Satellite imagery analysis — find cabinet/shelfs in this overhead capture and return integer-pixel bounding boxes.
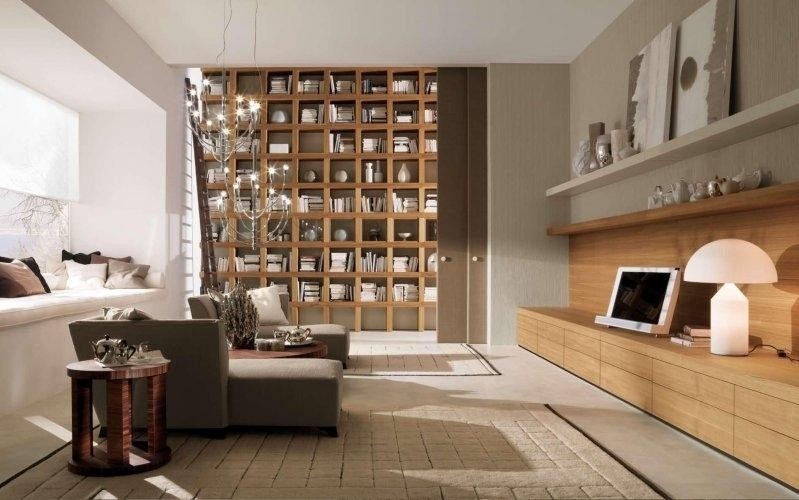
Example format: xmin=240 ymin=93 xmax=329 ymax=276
xmin=197 ymin=68 xmax=436 ymax=335
xmin=515 ymin=184 xmax=799 ymax=495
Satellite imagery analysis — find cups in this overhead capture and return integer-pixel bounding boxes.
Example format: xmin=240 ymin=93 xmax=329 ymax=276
xmin=137 ymin=342 xmax=152 ymax=361
xmin=257 ymin=339 xmax=284 ymax=352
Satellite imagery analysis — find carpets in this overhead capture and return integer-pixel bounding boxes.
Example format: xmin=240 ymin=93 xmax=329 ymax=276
xmin=336 ymin=338 xmax=502 ymax=377
xmin=0 ymin=402 xmax=663 ymax=499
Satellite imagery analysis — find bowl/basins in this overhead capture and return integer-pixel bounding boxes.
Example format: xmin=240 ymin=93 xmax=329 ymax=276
xmin=398 ymin=233 xmax=412 ymax=238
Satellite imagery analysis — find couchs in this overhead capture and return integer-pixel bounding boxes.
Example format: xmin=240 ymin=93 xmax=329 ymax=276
xmin=188 ymin=294 xmax=350 ymax=369
xmin=70 ymin=308 xmax=345 ymax=436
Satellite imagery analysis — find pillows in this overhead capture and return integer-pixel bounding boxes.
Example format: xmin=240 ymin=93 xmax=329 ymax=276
xmin=0 ymin=250 xmax=163 ymax=299
xmin=246 ymin=285 xmax=289 ymax=325
xmin=101 ymin=308 xmax=150 ymax=320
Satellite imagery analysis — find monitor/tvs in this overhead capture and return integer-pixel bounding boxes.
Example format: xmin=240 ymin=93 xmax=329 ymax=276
xmin=594 ymin=267 xmax=682 ymax=338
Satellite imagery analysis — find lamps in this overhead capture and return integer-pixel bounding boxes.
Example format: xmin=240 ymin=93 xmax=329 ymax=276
xmin=683 ymin=237 xmax=778 ymax=355
xmin=182 ymin=1 xmax=293 ymax=250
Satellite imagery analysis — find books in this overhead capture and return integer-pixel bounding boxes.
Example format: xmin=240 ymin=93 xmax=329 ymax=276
xmin=201 ymin=134 xmax=260 ymax=153
xmin=240 ymin=103 xmax=436 ymax=123
xmin=209 ymin=76 xmax=436 ymax=93
xmin=207 ymin=168 xmax=260 ymax=181
xmin=217 ymin=252 xmax=417 ymax=272
xmin=209 ymin=192 xmax=436 ymax=213
xmin=682 ymin=324 xmax=711 ymax=338
xmin=211 ymin=218 xmax=229 ymax=242
xmin=225 ymin=281 xmax=436 ymax=301
xmin=329 ymin=133 xmax=436 ymax=152
xmin=676 ymin=333 xmax=711 ymax=342
xmin=670 ymin=336 xmax=711 ymax=348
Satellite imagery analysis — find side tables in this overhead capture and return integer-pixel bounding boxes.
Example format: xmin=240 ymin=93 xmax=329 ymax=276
xmin=65 ymin=349 xmax=172 ymax=476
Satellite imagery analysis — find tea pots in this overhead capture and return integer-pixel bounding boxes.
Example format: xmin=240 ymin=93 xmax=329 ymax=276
xmin=89 ymin=336 xmax=133 ymax=365
xmin=647 ymin=168 xmax=761 ymax=211
xmin=272 ymin=326 xmax=311 ymax=344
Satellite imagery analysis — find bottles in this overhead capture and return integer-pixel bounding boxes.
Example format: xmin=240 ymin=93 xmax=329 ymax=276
xmin=571 ymin=127 xmax=632 ymax=178
xmin=365 ymin=160 xmax=384 ymax=182
xmin=268 ymin=230 xmax=291 ymax=242
xmin=397 ymin=163 xmax=412 ymax=182
xmin=328 ymin=104 xmax=336 ymax=123
